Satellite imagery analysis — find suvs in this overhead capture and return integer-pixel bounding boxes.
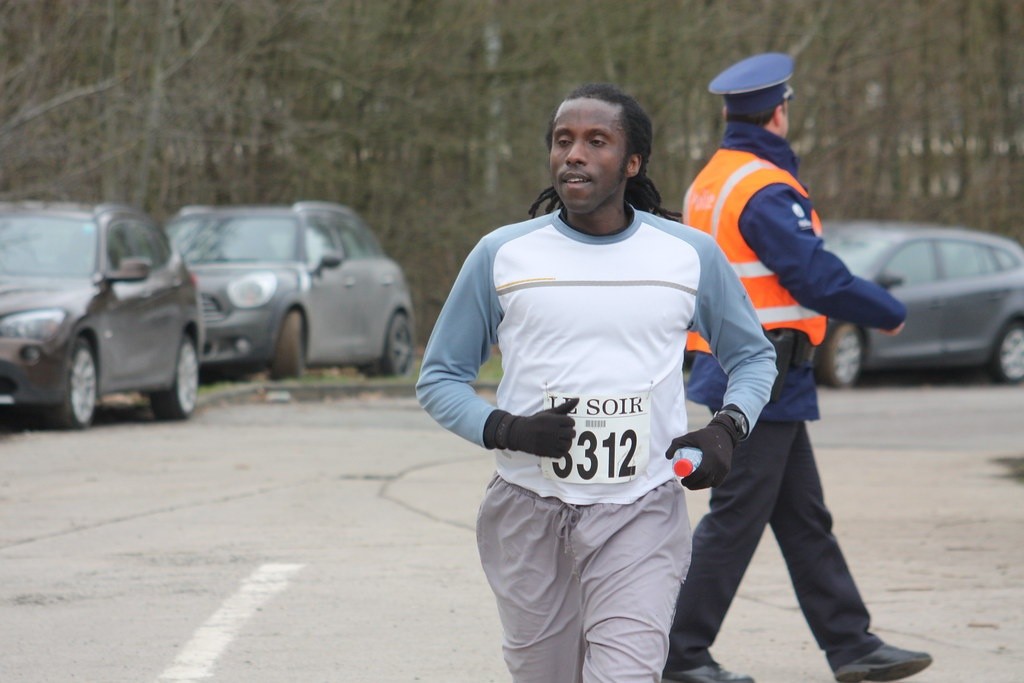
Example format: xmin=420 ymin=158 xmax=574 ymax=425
xmin=155 ymin=200 xmax=420 ymax=385
xmin=0 ymin=201 xmax=209 ymax=430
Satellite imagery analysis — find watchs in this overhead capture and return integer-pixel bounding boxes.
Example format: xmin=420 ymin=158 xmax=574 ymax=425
xmin=713 ymin=410 xmax=748 ymax=439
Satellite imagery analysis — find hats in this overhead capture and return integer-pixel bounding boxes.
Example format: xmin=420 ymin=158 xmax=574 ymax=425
xmin=708 ymin=53 xmax=795 ymax=113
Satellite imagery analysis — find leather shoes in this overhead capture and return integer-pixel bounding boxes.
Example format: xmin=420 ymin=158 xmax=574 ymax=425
xmin=834 ymin=643 xmax=932 ymax=683
xmin=662 ymin=663 xmax=754 ymax=683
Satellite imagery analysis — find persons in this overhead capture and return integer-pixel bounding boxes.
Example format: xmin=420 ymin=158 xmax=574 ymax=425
xmin=415 ymin=78 xmax=780 ymax=683
xmin=663 ymin=53 xmax=934 ymax=683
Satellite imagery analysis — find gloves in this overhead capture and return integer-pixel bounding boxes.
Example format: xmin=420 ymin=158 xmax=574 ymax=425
xmin=665 ymin=414 xmax=739 ymax=491
xmin=485 ymin=398 xmax=579 ymax=459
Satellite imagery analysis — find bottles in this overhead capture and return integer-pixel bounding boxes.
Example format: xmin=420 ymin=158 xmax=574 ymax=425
xmin=671 ymin=447 xmax=704 ymax=479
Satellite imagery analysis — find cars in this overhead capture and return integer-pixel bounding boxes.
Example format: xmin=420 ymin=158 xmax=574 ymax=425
xmin=808 ymin=223 xmax=1024 ymax=386
